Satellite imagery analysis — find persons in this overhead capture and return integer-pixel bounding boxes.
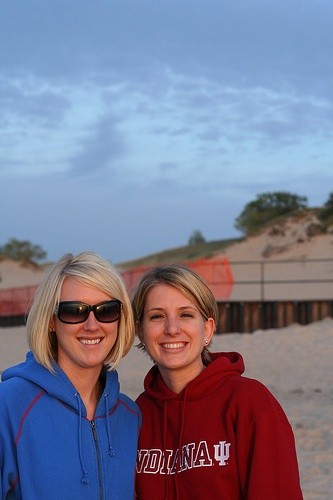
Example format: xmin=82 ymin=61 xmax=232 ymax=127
xmin=0 ymin=251 xmax=143 ymax=500
xmin=130 ymin=265 xmax=303 ymax=500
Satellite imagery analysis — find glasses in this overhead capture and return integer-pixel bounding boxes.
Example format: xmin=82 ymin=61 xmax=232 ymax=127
xmin=54 ymin=299 xmax=120 ymax=324
